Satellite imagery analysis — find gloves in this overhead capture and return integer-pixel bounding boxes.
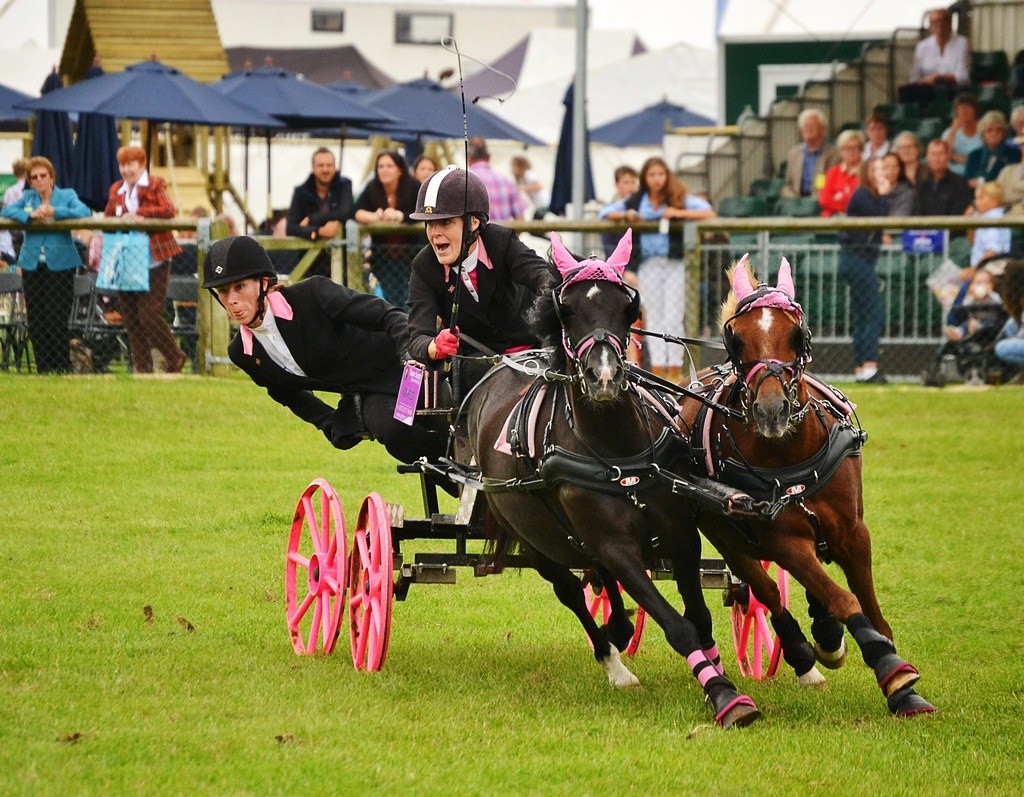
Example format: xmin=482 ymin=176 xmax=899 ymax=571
xmin=434 ymin=326 xmax=460 ymax=359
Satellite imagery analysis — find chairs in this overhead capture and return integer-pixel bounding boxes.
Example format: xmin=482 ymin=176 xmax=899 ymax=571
xmin=0 ymin=233 xmax=202 ymax=373
xmin=719 ymin=50 xmax=1024 ymax=327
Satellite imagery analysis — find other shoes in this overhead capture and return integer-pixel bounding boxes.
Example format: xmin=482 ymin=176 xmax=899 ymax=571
xmin=994 ymin=364 xmax=1023 ymax=387
xmin=854 ymin=365 xmax=878 ymax=382
xmin=168 ymin=351 xmax=188 ymax=372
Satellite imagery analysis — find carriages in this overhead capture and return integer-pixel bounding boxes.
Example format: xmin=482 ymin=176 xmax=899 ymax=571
xmin=283 ymin=228 xmax=941 ymax=733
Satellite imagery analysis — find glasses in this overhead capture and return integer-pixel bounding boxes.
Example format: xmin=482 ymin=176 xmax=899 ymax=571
xmin=30 ymin=173 xmax=49 ymax=180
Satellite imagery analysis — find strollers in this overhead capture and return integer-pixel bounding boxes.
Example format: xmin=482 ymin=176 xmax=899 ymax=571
xmin=920 ymin=251 xmax=1024 ymax=388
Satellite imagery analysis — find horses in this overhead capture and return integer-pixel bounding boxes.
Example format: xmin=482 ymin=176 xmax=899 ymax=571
xmin=465 ymin=227 xmax=814 ymax=732
xmin=673 ymin=251 xmax=937 ymax=716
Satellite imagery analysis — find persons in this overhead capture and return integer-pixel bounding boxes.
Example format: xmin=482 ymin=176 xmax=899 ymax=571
xmin=782 ymin=9 xmax=1024 ymax=388
xmin=406 ymin=165 xmax=558 ymax=397
xmin=601 ymin=156 xmax=715 ymax=380
xmin=257 ymin=137 xmax=546 ymax=307
xmin=0 ymin=155 xmax=93 ymax=374
xmin=100 ymin=145 xmax=187 ymax=372
xmin=201 ymin=235 xmax=463 ymax=497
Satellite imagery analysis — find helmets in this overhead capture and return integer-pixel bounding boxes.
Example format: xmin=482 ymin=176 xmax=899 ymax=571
xmin=200 ymin=235 xmax=277 ymax=289
xmin=410 ymin=163 xmax=490 ymax=222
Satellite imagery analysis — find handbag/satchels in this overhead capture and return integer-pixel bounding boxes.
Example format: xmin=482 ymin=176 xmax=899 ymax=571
xmin=95 ymin=229 xmax=153 ymax=294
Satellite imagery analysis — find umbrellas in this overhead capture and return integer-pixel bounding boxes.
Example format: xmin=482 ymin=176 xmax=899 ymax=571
xmin=1 ymin=54 xmax=720 ymax=216
